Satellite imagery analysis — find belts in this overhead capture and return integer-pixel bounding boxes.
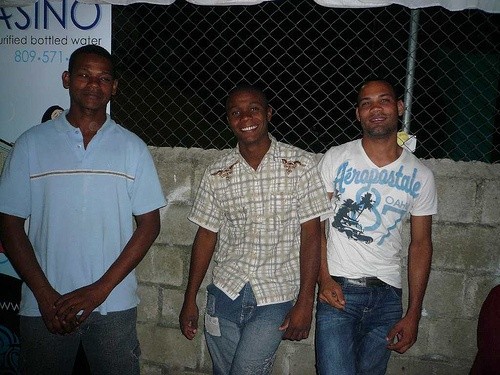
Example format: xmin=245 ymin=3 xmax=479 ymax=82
xmin=331 ymin=275 xmax=387 ymax=287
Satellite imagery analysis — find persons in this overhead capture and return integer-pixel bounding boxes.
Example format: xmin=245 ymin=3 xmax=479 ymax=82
xmin=0 ymin=44 xmax=167 ymax=375
xmin=179 ymin=84 xmax=334 ymax=375
xmin=316 ymin=81 xmax=438 ymax=375
